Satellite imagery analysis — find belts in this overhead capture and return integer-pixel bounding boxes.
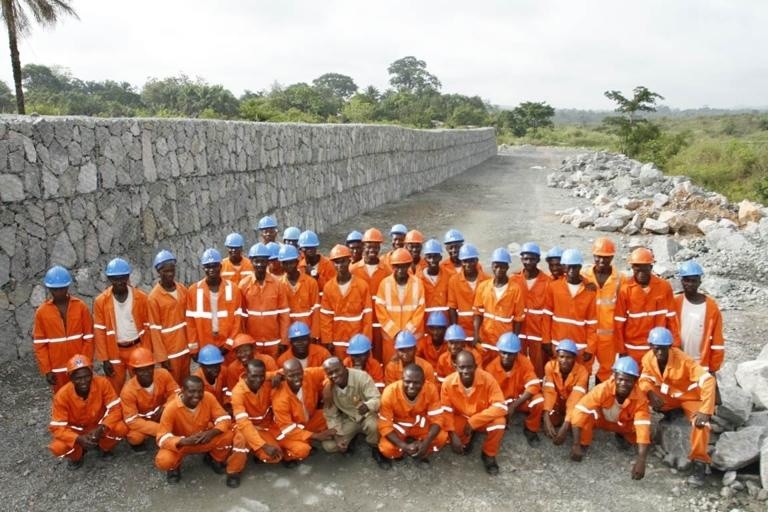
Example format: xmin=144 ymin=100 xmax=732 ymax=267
xmin=118 ymin=337 xmax=140 ymax=348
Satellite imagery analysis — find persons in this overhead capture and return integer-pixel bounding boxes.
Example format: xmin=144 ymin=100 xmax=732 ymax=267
xmin=32 ymin=215 xmax=727 ymax=492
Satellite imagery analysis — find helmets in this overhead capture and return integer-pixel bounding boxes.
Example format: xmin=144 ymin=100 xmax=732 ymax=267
xmin=66 ymin=354 xmax=94 ymax=377
xmin=329 ymin=224 xmax=480 ymax=265
xmin=496 ymin=331 xmax=521 ymax=354
xmin=628 ymin=247 xmax=655 ymax=265
xmin=224 ymin=233 xmax=244 ymax=248
xmin=43 ymin=265 xmax=73 ymax=289
xmin=346 ymin=334 xmax=371 ymax=355
xmin=647 ymin=326 xmax=674 ymax=346
xmin=491 ymin=248 xmax=512 ymax=263
xmin=105 ymin=258 xmax=131 ymax=276
xmin=592 ymin=237 xmax=615 ymax=257
xmin=198 ymin=344 xmax=225 ymax=366
xmin=611 ymin=356 xmax=640 ymax=378
xmin=231 ymin=332 xmax=256 ymax=352
xmin=679 ymin=262 xmax=703 ymax=277
xmin=200 ymin=248 xmax=222 ymax=265
xmin=129 ymin=347 xmax=155 ymax=368
xmin=153 ymin=249 xmax=176 ymax=270
xmin=520 ymin=242 xmax=584 ymax=265
xmin=394 ymin=311 xmax=466 ymax=349
xmin=555 ymin=338 xmax=579 ymax=357
xmin=248 ymin=216 xmax=320 ymax=261
xmin=287 ymin=320 xmax=310 ymax=339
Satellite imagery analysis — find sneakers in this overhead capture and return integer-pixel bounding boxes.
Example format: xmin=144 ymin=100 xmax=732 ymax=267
xmin=166 ymin=467 xmax=180 ymax=483
xmin=67 ymin=455 xmax=83 ymax=470
xmin=615 ymin=432 xmax=638 ymax=455
xmin=463 ymin=430 xmax=475 ymax=456
xmin=95 ymin=444 xmax=114 ymax=460
xmin=523 ymin=427 xmax=540 ymax=448
xmin=342 ymin=438 xmax=355 ymax=459
xmin=227 ymin=472 xmax=241 ymax=488
xmin=481 ymin=451 xmax=499 ymax=475
xmin=687 ymin=460 xmax=713 ymax=487
xmin=130 ymin=443 xmax=148 ymax=455
xmin=371 ymin=446 xmax=392 ymax=470
xmin=215 ymin=462 xmax=226 ymax=474
xmin=280 ymin=459 xmax=297 ymax=468
